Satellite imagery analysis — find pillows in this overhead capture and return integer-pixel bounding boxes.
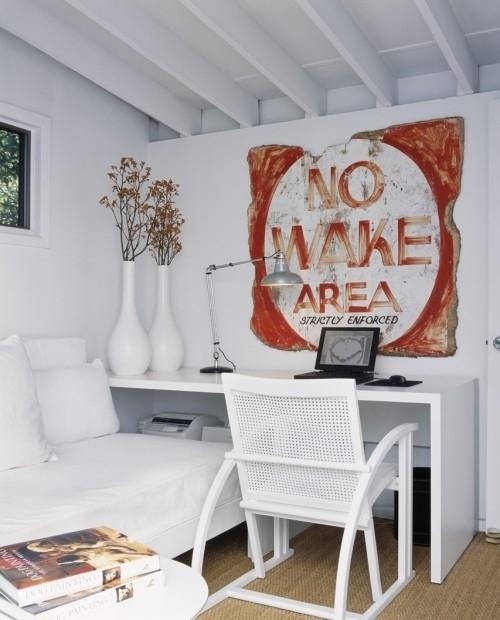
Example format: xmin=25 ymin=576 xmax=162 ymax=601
xmin=34 ymin=359 xmax=119 ymax=445
xmin=23 ymin=338 xmax=86 ymax=369
xmin=0 ymin=334 xmax=57 ymax=471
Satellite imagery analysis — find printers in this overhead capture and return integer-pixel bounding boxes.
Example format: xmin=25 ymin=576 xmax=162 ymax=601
xmin=137 ymin=411 xmax=225 ymax=441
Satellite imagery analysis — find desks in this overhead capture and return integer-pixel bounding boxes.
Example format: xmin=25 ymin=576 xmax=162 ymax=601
xmin=108 ymin=369 xmax=478 ymax=584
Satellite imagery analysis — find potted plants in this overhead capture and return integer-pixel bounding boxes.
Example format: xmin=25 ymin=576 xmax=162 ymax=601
xmin=142 ymin=177 xmax=183 ymax=371
xmin=100 ymin=157 xmax=151 ymax=377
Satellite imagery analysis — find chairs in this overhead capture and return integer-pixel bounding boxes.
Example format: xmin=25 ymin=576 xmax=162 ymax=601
xmin=191 ymin=372 xmax=417 ymax=620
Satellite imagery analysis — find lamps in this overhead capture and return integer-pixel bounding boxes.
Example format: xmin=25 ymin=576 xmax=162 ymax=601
xmin=199 ymin=249 xmax=303 ymax=375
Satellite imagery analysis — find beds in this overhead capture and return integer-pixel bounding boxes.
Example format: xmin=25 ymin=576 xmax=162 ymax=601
xmin=0 ymin=433 xmax=245 ymax=559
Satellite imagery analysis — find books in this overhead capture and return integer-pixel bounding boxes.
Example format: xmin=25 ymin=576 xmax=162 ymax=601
xmin=0 ymin=524 xmax=164 ymax=620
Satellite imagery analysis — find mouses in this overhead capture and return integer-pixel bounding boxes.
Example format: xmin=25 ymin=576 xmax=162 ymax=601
xmin=388 ymin=375 xmax=407 ymax=385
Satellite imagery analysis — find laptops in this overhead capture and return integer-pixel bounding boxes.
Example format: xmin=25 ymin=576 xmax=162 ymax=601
xmin=294 ymin=326 xmax=380 ymax=385
